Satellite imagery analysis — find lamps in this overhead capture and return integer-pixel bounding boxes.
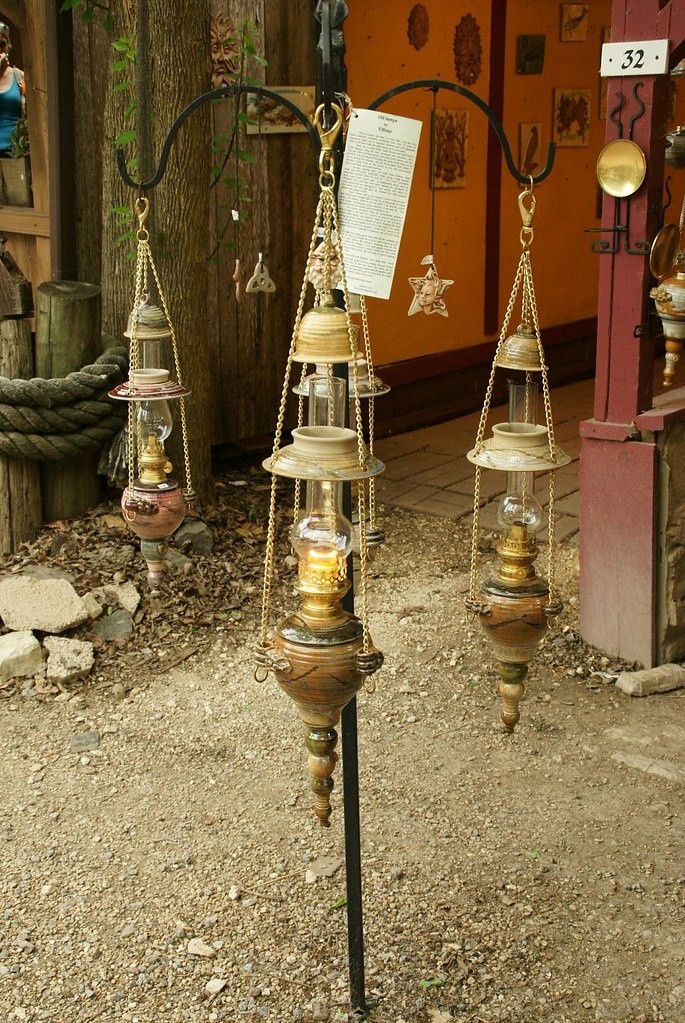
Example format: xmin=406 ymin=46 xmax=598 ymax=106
xmin=252 ymin=99 xmax=386 ymax=829
xmin=107 ymin=179 xmax=194 ymax=600
xmin=461 ymin=172 xmax=570 ymax=738
